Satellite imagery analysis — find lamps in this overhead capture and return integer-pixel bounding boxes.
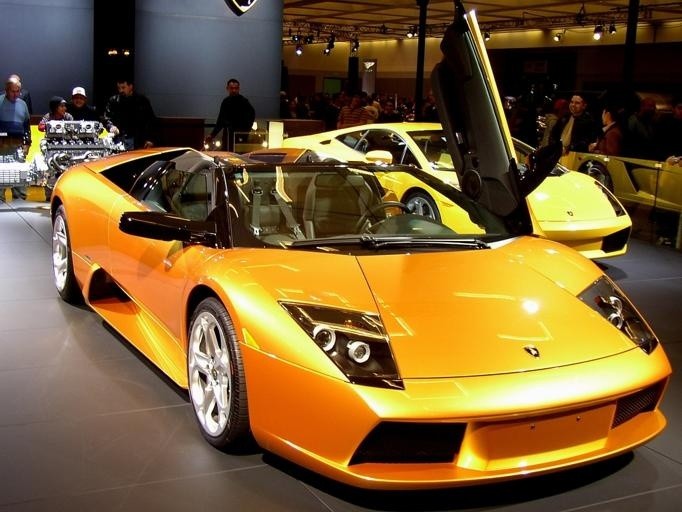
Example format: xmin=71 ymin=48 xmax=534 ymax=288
xmin=292 ymin=25 xmax=418 ymax=55
xmin=592 ymin=24 xmax=617 ymax=41
xmin=483 ymin=32 xmax=490 ymax=41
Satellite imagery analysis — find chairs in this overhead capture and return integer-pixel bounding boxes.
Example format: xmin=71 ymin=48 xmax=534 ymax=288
xmin=169 ymin=159 xmax=373 ymax=247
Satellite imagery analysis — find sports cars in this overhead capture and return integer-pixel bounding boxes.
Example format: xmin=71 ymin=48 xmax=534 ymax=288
xmin=50 ymin=9 xmax=675 ymax=495
xmin=247 ymin=122 xmax=633 ymax=263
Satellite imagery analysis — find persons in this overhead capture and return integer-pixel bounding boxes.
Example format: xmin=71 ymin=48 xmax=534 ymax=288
xmin=0 ymin=79 xmax=31 ymax=202
xmin=279 ymin=87 xmax=440 ymax=129
xmin=0 ymin=74 xmax=34 ymax=115
xmin=65 ymin=86 xmax=102 ymax=122
xmin=102 ymin=76 xmax=155 ymax=149
xmin=205 ymin=78 xmax=255 ymax=153
xmin=504 ymin=79 xmax=682 ymax=249
xmin=39 ymin=95 xmax=74 ymax=200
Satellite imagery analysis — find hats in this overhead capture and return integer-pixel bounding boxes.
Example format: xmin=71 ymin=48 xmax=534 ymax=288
xmin=71 ymin=86 xmax=88 ymax=98
xmin=49 ymin=95 xmax=67 ymax=113
